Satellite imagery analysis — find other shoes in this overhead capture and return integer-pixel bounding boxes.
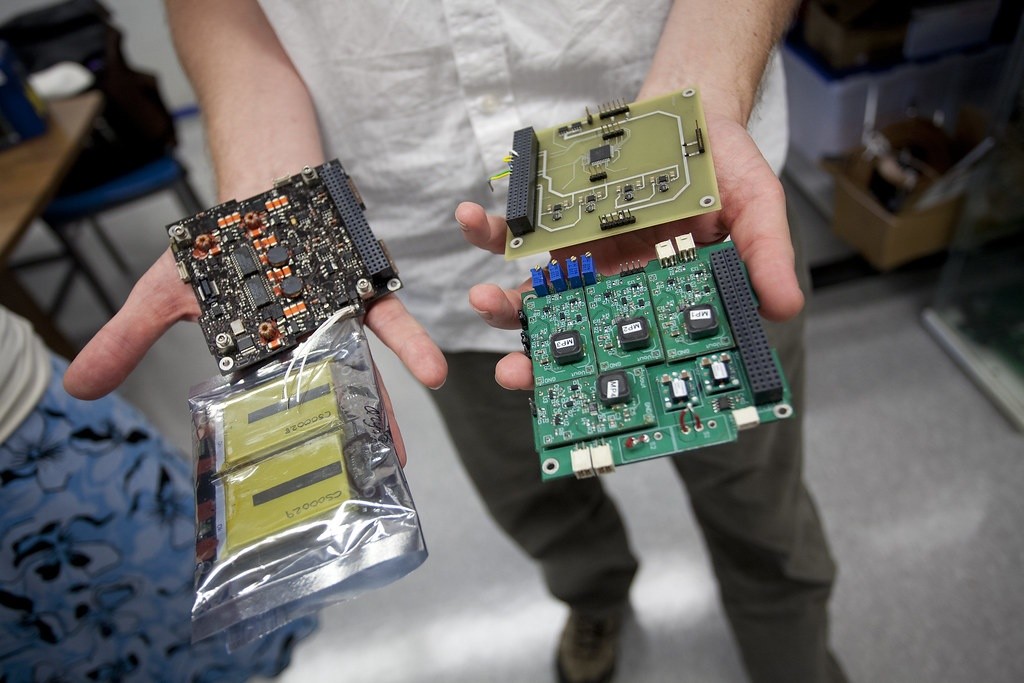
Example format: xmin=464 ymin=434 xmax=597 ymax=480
xmin=556 ymin=604 xmax=622 ymax=683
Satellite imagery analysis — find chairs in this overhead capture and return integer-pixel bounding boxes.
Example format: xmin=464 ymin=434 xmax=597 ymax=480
xmin=0 ymin=0 xmax=208 ymax=320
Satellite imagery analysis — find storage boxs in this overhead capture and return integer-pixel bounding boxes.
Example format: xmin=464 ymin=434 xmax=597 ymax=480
xmin=781 ymin=0 xmax=1024 ymax=271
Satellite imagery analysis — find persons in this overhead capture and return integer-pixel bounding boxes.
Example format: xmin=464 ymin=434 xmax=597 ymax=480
xmin=0 ymin=306 xmax=322 ymax=683
xmin=63 ymin=0 xmax=854 ymax=683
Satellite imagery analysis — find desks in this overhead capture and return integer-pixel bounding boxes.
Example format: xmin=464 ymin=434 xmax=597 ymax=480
xmin=0 ymin=84 xmax=117 ymax=362
